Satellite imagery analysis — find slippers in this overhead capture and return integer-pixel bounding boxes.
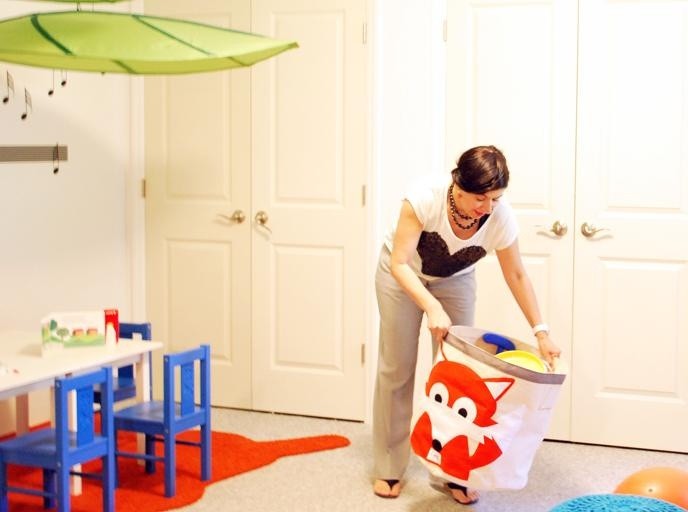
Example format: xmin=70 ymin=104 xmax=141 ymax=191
xmin=372 ymin=476 xmax=401 ymax=498
xmin=442 ymin=481 xmax=480 ymax=505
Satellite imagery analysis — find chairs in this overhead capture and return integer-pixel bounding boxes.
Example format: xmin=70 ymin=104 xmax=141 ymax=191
xmin=1 ymin=362 xmax=116 ymax=512
xmin=115 ymin=344 xmax=210 ymax=498
xmin=94 ymin=323 xmax=152 ymax=403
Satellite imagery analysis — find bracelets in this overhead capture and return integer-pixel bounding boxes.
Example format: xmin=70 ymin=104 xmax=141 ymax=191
xmin=532 ymin=323 xmax=551 ymax=336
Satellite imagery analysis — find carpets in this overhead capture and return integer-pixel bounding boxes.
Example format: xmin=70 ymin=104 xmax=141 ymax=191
xmin=0 ymin=410 xmax=351 ymax=512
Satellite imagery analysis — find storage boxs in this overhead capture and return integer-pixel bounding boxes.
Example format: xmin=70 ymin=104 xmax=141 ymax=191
xmin=411 ymin=326 xmax=567 ymax=491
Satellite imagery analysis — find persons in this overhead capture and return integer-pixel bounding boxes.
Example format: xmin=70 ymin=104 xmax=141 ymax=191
xmin=372 ymin=144 xmax=562 ymax=505
